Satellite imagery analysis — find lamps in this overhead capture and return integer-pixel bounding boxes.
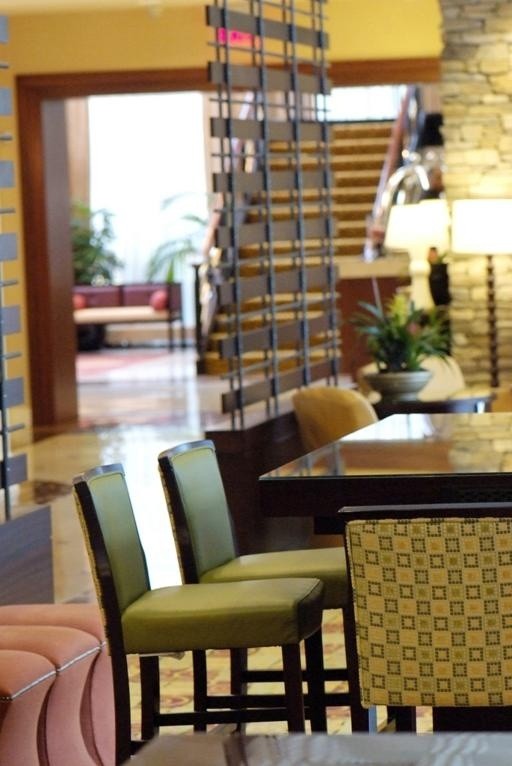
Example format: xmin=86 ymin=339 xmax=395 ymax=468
xmin=380 ymin=195 xmax=512 ymax=388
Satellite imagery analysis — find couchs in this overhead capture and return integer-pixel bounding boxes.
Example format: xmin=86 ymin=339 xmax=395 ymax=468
xmin=71 ymin=280 xmax=189 ymax=354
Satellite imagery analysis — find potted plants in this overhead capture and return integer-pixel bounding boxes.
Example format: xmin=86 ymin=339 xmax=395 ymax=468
xmin=342 ymin=294 xmax=454 ymax=403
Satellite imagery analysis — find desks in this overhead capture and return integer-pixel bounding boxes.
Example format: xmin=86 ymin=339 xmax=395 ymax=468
xmin=115 ymin=724 xmax=512 ymax=765
